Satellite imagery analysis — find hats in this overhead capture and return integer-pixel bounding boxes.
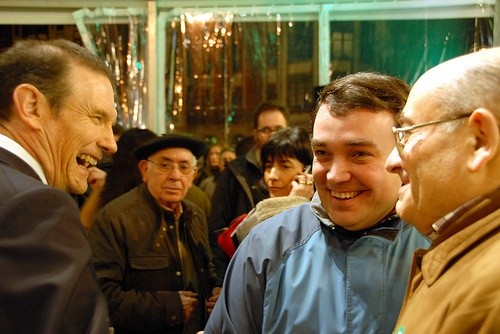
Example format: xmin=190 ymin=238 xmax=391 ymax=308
xmin=134 ymin=134 xmax=206 ymax=160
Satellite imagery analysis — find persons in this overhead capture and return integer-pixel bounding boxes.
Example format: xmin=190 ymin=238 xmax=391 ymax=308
xmin=204 ymin=72 xmax=432 ymax=334
xmin=70 ymin=102 xmax=317 ymax=334
xmin=385 ymin=46 xmax=500 ymax=334
xmin=0 ymin=38 xmax=118 ymax=334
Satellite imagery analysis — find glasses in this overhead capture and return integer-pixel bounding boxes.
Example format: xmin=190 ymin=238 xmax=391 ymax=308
xmin=392 ymin=113 xmax=471 ymax=159
xmin=147 ymin=159 xmax=196 ymax=173
xmin=257 ymin=127 xmax=281 ymax=134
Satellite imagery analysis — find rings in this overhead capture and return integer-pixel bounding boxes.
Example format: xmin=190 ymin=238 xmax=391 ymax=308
xmin=299 ymin=179 xmax=314 ymax=185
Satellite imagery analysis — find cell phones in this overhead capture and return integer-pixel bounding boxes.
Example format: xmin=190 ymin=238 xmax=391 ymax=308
xmin=298 ymin=164 xmax=312 ymax=183
